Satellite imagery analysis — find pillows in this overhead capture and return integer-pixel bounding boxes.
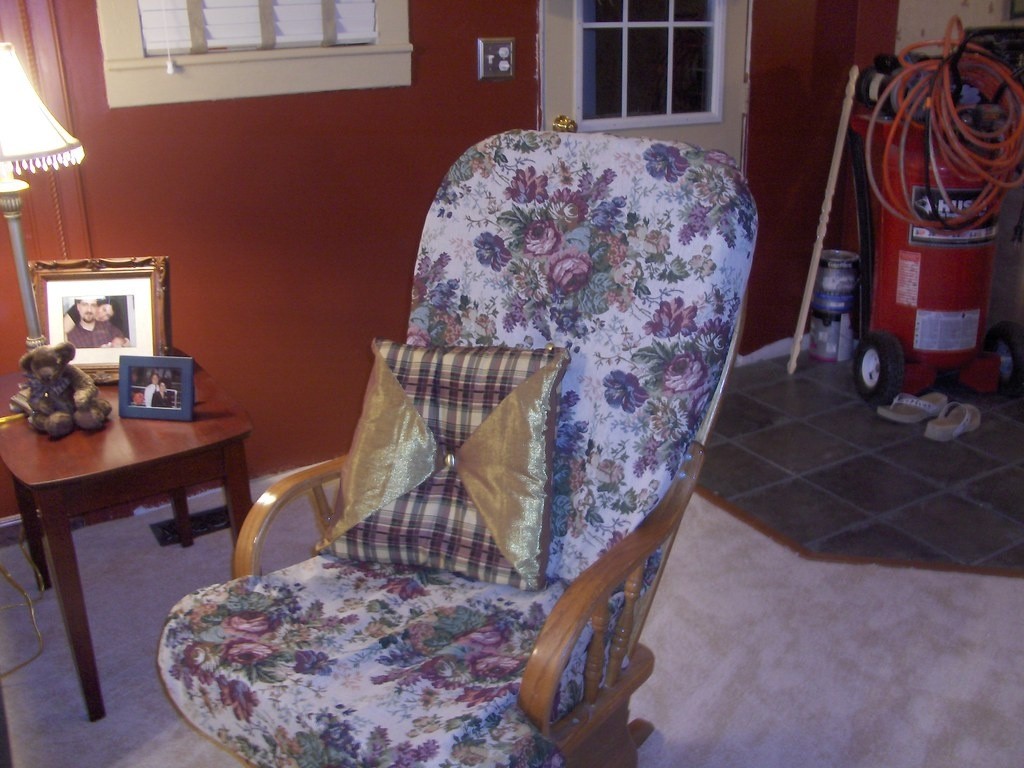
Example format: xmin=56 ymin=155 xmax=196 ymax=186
xmin=314 ymin=335 xmax=571 ymax=588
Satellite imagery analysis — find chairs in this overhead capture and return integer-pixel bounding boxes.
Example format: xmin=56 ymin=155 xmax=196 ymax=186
xmin=156 ymin=129 xmax=748 ymax=768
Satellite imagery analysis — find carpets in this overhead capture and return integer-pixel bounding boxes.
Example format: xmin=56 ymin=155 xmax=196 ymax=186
xmin=2 ymin=458 xmax=1024 ymax=768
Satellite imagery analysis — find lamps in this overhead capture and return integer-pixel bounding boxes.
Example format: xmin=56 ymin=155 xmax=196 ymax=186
xmin=0 ymin=42 xmax=85 ymax=350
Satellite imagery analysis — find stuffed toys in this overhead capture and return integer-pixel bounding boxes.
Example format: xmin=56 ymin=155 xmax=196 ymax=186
xmin=19 ymin=343 xmax=112 ymax=436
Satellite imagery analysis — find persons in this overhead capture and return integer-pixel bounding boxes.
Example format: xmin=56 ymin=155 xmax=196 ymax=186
xmin=62 ymin=297 xmax=129 ymax=347
xmin=129 ymin=366 xmax=174 ymax=408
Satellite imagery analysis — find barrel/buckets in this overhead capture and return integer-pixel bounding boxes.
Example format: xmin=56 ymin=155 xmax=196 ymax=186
xmin=809 ymin=249 xmax=861 ymax=363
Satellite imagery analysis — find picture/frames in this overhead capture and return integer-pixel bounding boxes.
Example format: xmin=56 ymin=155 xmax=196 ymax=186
xmin=478 ymin=38 xmax=515 ymax=82
xmin=28 ymin=256 xmax=169 ymax=385
xmin=118 ymin=355 xmax=194 ymax=421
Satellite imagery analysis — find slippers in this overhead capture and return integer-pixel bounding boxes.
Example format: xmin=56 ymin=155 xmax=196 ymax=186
xmin=877 ymin=392 xmax=948 ymax=424
xmin=924 ymin=402 xmax=982 ymax=441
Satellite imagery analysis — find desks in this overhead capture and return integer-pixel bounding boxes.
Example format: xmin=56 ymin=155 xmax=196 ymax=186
xmin=0 ymin=345 xmax=253 ymax=724
